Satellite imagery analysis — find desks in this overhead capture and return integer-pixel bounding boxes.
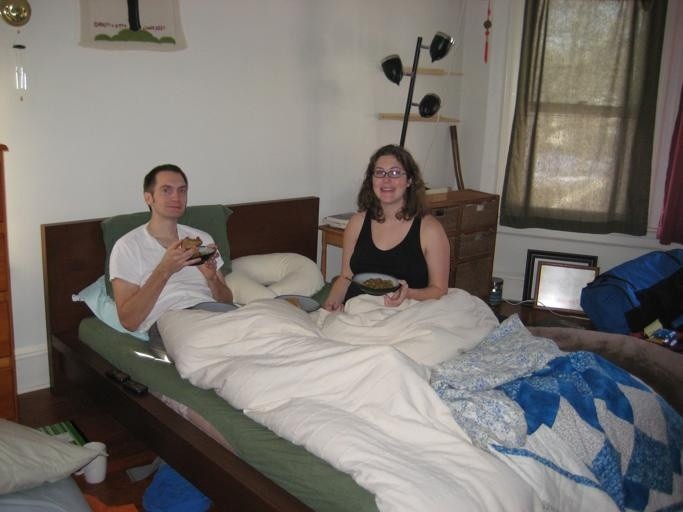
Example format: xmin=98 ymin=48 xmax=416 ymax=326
xmin=318 ymin=221 xmax=345 ymax=284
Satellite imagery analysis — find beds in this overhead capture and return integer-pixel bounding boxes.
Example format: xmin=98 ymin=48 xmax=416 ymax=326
xmin=39 ymin=196 xmax=683 ymax=511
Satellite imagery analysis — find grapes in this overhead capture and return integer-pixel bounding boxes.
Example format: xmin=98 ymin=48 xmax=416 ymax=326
xmin=362 ymin=278 xmax=394 ymax=291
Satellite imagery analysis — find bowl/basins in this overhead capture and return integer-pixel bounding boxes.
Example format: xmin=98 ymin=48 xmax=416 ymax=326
xmin=351 ymin=273 xmax=400 ymax=296
xmin=187 ymin=246 xmax=215 ymax=267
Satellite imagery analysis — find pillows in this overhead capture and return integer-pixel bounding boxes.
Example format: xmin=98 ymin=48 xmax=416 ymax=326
xmin=70 ymin=272 xmax=151 ymax=342
xmin=0 ymin=418 xmax=108 ymax=500
xmin=225 ymin=252 xmax=326 ymax=307
xmin=100 ymin=203 xmax=234 ymax=301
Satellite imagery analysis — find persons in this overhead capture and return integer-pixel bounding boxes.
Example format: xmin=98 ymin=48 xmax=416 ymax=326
xmin=109 ymin=164 xmax=237 ymax=348
xmin=322 ymin=144 xmax=450 ymax=312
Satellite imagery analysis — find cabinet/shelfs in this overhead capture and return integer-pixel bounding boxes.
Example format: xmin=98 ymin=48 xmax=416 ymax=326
xmin=0 ymin=144 xmax=19 ymax=419
xmin=423 ymin=188 xmax=499 ymax=304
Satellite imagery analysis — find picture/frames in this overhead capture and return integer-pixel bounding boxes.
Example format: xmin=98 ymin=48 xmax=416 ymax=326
xmin=522 ymin=248 xmax=598 ymax=309
xmin=532 ymin=260 xmax=600 ymax=316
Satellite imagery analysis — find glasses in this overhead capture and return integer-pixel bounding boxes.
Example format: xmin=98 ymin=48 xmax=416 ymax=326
xmin=373 ymin=168 xmax=407 ymax=178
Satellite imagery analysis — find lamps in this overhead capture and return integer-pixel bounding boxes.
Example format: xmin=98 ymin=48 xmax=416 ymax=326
xmin=376 ymin=30 xmax=460 ymax=149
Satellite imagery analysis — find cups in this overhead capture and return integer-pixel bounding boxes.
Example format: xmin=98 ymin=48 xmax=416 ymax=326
xmin=71 ymin=441 xmax=108 ymax=485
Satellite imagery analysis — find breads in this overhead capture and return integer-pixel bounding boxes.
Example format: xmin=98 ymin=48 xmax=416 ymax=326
xmin=181 ymin=236 xmax=202 ymax=250
xmin=287 ymin=297 xmax=302 ymax=310
